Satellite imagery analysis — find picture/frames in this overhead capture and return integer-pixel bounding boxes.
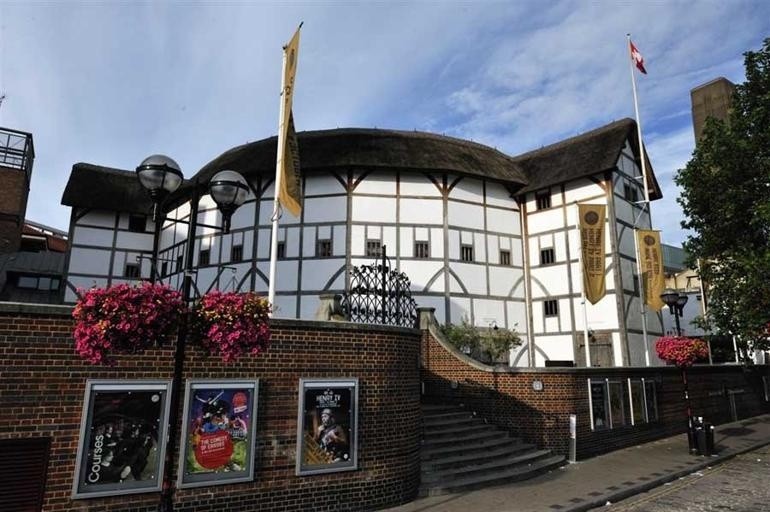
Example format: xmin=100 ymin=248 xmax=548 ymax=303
xmin=293 ymin=376 xmax=359 ymax=477
xmin=70 ymin=376 xmax=263 ymax=500
xmin=587 ymin=376 xmax=660 ymax=432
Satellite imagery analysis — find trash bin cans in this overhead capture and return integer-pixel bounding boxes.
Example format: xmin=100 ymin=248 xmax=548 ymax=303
xmin=693 ymin=421 xmax=713 ymax=456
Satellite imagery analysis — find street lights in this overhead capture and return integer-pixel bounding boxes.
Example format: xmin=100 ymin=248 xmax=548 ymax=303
xmin=660 ymin=288 xmax=699 ymax=454
xmin=137 ymin=154 xmax=250 ymax=511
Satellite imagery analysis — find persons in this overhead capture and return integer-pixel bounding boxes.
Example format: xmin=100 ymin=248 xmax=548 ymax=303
xmin=85 ymin=422 xmax=152 ymax=484
xmin=312 ymin=409 xmax=349 ymax=460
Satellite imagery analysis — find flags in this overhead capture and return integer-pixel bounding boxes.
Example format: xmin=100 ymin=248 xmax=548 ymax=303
xmin=631 ymin=40 xmax=647 ymax=74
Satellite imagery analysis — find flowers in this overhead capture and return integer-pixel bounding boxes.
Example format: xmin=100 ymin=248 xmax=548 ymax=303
xmin=653 ymin=332 xmax=710 ymax=370
xmin=70 ymin=279 xmax=271 ymax=371
